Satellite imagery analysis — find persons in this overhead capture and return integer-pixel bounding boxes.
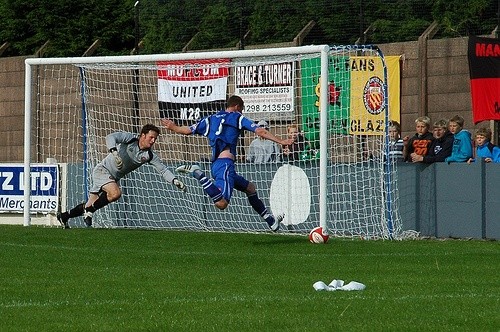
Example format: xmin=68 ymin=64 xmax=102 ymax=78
xmin=162 ymin=95 xmax=296 ymax=232
xmin=467 ymin=126 xmax=500 ymax=163
xmin=402 ymin=116 xmax=434 ymax=162
xmin=57 ymin=124 xmax=187 ymax=230
xmin=246 ymin=120 xmax=280 ymax=162
xmin=374 ymin=121 xmax=404 ymax=162
xmin=444 ymin=115 xmax=473 ymax=162
xmin=279 ymin=124 xmax=314 ymax=163
xmin=412 ymin=119 xmax=454 ymax=163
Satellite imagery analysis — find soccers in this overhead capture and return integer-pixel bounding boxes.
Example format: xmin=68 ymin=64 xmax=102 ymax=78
xmin=309 ymin=226 xmax=329 ymax=244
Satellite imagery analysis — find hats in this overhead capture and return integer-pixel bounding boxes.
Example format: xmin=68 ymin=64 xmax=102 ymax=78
xmin=257 ymin=120 xmax=270 ymax=130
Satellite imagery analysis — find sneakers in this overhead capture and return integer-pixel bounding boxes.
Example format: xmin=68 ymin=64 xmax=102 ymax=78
xmin=56 ymin=211 xmax=71 ymax=229
xmin=82 ymin=203 xmax=94 ymax=228
xmin=174 ymin=164 xmax=202 ymax=176
xmin=269 ymin=210 xmax=285 ymax=233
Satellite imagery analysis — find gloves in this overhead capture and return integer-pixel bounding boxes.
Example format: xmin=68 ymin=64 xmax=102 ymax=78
xmin=111 ymin=150 xmax=123 ymax=170
xmin=172 ymin=179 xmax=188 ymax=193
